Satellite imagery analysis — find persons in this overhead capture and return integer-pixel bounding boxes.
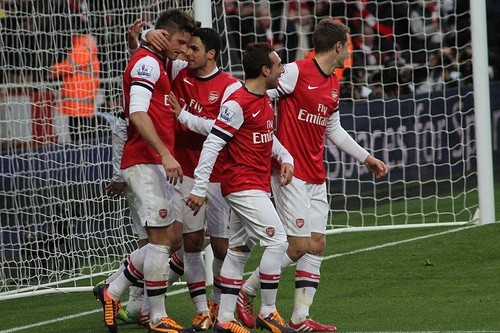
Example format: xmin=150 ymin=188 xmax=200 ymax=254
xmin=93 ymin=105 xmax=149 ymax=322
xmin=186 ymin=42 xmax=296 ymax=333
xmin=0 ymin=0 xmax=500 ymax=106
xmin=97 ymin=10 xmax=198 ymax=333
xmin=47 ymin=20 xmax=99 ymax=141
xmin=236 ymin=19 xmax=389 ymax=333
xmin=127 ymin=18 xmax=245 ymax=330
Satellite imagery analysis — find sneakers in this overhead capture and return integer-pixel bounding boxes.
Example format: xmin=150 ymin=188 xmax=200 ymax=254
xmin=289 ymin=318 xmax=337 ymax=333
xmin=149 ymin=316 xmax=184 ymax=333
xmin=213 ymin=317 xmax=250 ymax=333
xmin=125 ymin=310 xmax=139 ymax=323
xmin=93 ymin=280 xmax=129 ymax=322
xmin=98 ymin=284 xmax=121 ymax=333
xmin=208 ymin=299 xmax=219 ymax=322
xmin=237 ymin=288 xmax=256 ymax=329
xmin=257 ymin=310 xmax=296 ymax=333
xmin=192 ymin=313 xmax=212 ymax=330
xmin=139 ymin=308 xmax=150 ymax=325
